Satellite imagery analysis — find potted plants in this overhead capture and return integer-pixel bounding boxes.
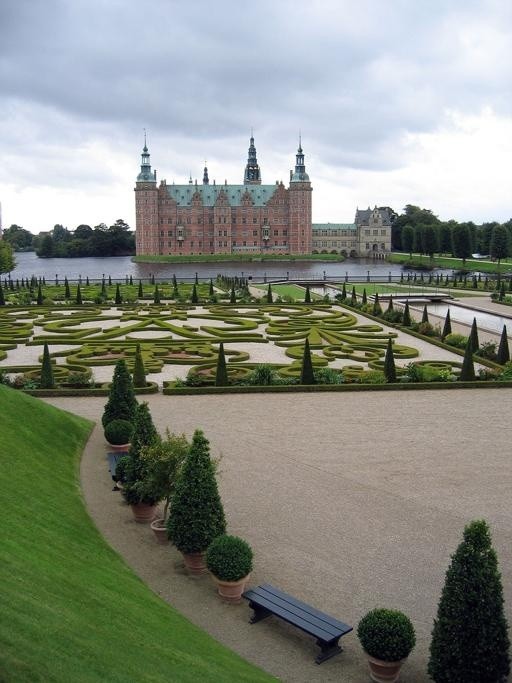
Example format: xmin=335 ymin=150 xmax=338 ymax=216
xmin=204 ymin=531 xmax=253 ymax=600
xmin=115 ymin=402 xmax=162 ymax=524
xmin=357 ymin=607 xmax=416 ymax=683
xmin=104 ymin=418 xmax=135 ymax=454
xmin=139 ymin=433 xmax=225 ymax=545
xmin=168 ymin=427 xmax=228 ymax=576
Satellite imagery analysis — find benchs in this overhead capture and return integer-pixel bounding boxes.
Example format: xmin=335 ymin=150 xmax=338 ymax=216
xmin=241 ymin=583 xmax=353 ymax=665
